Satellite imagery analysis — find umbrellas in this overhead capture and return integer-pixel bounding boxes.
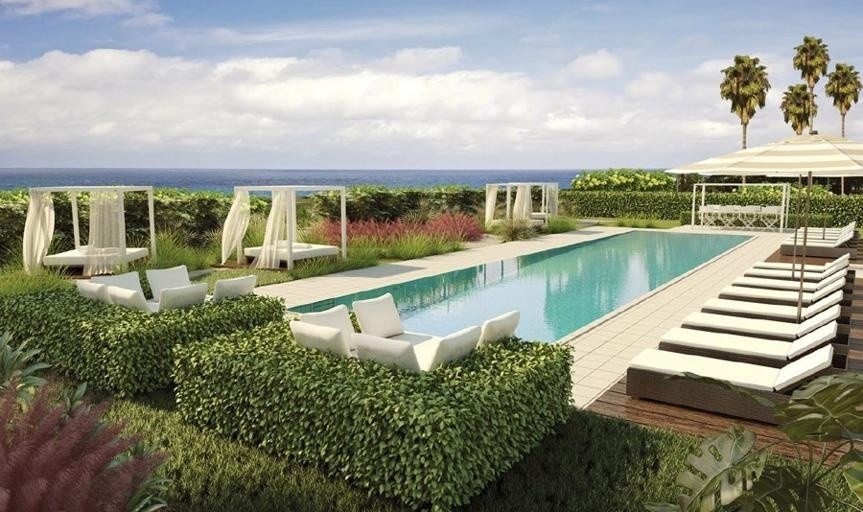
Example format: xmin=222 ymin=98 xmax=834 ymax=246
xmin=664 ymin=127 xmax=863 ymax=323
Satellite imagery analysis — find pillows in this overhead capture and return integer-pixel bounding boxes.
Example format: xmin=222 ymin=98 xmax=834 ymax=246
xmin=289 ymin=290 xmax=520 ymax=374
xmin=76 ymin=245 xmax=120 ymax=256
xmin=75 ymin=264 xmax=257 ymax=310
xmin=272 ymin=239 xmax=311 ymax=249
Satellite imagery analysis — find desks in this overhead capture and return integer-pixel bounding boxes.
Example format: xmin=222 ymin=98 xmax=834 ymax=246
xmin=697 ymin=210 xmax=781 ymax=232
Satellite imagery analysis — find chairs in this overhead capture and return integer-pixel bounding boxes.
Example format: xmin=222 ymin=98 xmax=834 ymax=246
xmin=659 ymin=321 xmax=838 ymax=370
xmin=627 ymin=344 xmax=835 ymax=429
xmin=780 ymin=221 xmax=857 ymax=259
xmin=752 ymin=253 xmax=851 ymax=273
xmin=681 ymin=304 xmax=841 ymax=341
xmin=717 ymin=276 xmax=846 ymax=308
xmin=743 ymin=260 xmax=849 ymax=282
xmin=701 ymin=290 xmax=844 ymax=324
xmin=732 ymin=268 xmax=848 ymax=293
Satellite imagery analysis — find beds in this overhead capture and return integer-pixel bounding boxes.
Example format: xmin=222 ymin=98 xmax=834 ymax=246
xmin=41 ymin=245 xmax=150 ymax=269
xmin=244 ymin=242 xmax=340 ymax=265
